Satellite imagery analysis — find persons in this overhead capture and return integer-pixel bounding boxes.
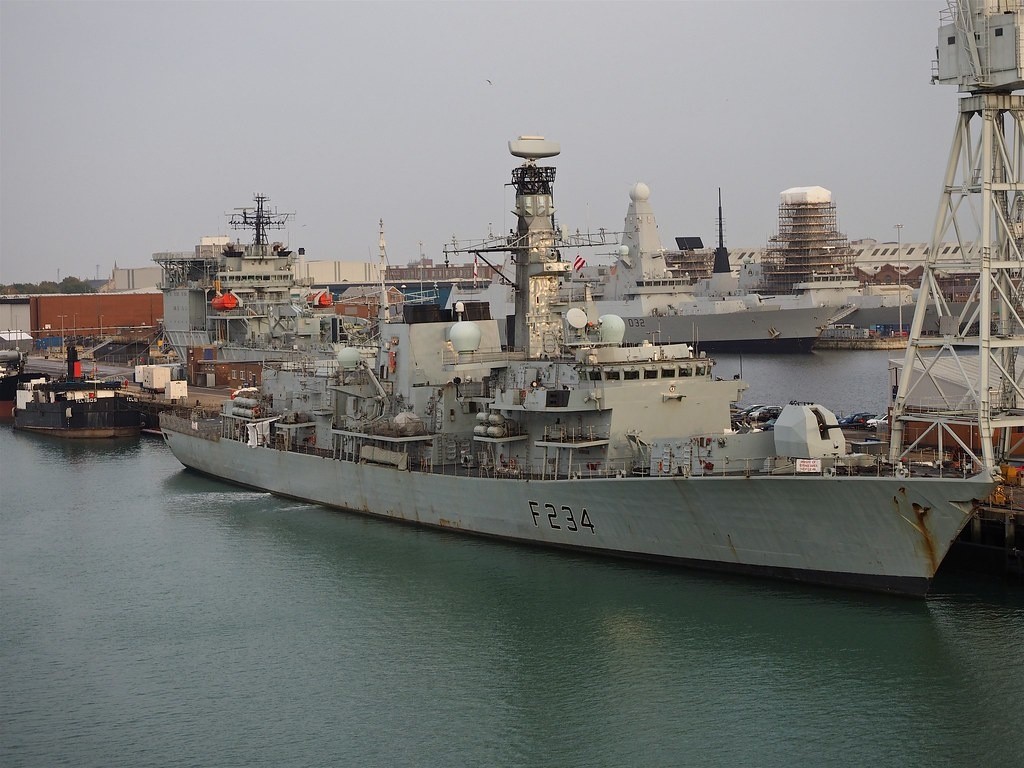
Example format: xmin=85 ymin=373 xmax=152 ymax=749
xmin=125 ymin=379 xmax=128 ymax=388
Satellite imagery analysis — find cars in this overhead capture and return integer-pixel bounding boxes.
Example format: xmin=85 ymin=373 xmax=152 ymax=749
xmin=838 ymin=412 xmax=887 ymax=428
xmin=736 ymin=404 xmax=783 ymax=421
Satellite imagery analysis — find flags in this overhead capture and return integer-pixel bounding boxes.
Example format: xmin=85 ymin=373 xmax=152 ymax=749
xmin=473 ymin=256 xmax=478 ymax=288
xmin=573 ymin=255 xmax=585 ymax=271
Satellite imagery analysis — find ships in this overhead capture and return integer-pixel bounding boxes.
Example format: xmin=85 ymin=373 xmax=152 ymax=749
xmin=159 ymin=134 xmax=997 ymax=597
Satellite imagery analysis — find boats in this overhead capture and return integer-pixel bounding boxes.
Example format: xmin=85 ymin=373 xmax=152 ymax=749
xmin=14 ymin=378 xmax=142 ymax=438
xmin=571 ymin=182 xmax=861 ymax=362
